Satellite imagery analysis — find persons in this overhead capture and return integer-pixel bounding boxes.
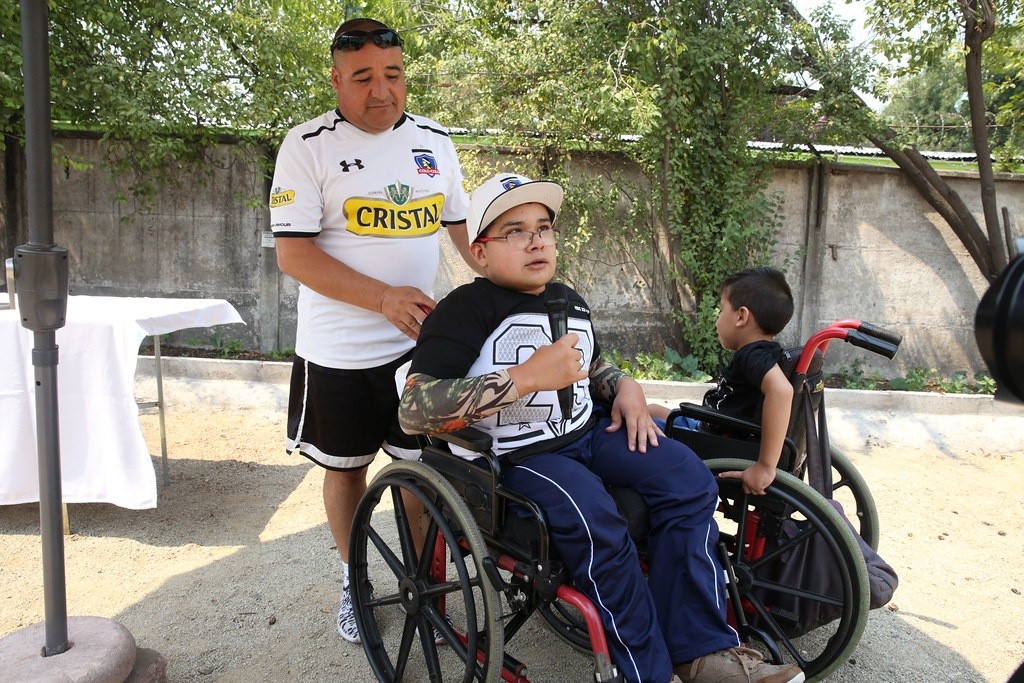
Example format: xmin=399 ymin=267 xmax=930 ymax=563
xmin=268 ymin=17 xmax=489 ymax=645
xmin=645 ymin=266 xmax=797 ymax=495
xmin=396 ymin=172 xmax=806 ymax=683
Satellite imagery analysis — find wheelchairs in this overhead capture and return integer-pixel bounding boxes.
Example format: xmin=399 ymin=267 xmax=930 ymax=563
xmin=661 ymin=320 xmax=903 ymax=681
xmin=345 ymin=402 xmax=784 ymax=683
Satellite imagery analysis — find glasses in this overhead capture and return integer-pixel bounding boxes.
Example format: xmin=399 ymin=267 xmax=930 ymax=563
xmin=331 ymin=28 xmax=405 ymax=53
xmin=477 ymin=230 xmax=561 ymax=248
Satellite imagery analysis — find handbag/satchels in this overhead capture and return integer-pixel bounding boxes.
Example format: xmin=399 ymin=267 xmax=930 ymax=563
xmin=744 ymin=385 xmax=901 ymax=640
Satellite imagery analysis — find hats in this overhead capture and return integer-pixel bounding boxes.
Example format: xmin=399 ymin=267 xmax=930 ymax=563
xmin=466 ymin=173 xmax=563 ymax=246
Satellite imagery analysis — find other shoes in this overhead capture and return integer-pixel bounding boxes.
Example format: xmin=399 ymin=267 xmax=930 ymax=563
xmin=336 ymin=581 xmax=375 ymax=644
xmin=415 ymin=615 xmax=453 ymax=645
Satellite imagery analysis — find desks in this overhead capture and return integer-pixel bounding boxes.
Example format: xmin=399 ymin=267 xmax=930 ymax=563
xmin=1 ymin=290 xmax=249 ymax=538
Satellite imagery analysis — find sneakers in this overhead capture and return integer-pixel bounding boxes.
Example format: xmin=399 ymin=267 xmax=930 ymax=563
xmin=680 ymin=644 xmax=805 ymax=683
